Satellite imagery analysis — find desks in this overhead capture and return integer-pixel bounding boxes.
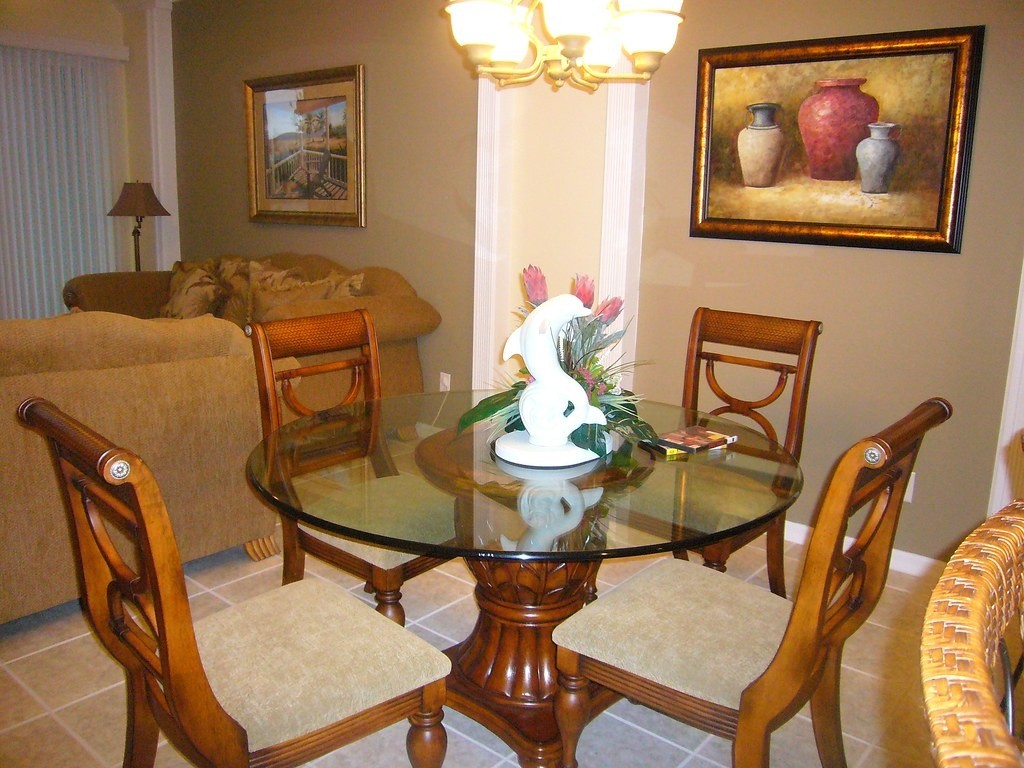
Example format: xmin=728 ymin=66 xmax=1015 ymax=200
xmin=247 ymin=397 xmax=803 ymax=768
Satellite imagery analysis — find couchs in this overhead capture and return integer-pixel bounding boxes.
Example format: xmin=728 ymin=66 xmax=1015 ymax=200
xmin=2 ymin=251 xmax=440 ymax=629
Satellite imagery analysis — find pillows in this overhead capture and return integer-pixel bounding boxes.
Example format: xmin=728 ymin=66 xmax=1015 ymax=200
xmin=155 ymin=255 xmax=364 ymax=329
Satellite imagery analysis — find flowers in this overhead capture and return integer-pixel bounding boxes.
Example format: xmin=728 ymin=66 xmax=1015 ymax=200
xmin=470 ymin=264 xmax=664 ymax=434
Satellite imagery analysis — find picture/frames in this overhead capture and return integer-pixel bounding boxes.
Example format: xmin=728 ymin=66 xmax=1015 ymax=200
xmin=242 ymin=64 xmax=366 ymax=228
xmin=688 ymin=23 xmax=988 ymax=255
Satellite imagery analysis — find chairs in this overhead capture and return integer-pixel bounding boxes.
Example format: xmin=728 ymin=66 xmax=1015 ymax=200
xmin=584 ymin=308 xmax=823 ymax=608
xmin=245 ymin=309 xmax=470 ymax=626
xmin=552 ymin=395 xmax=954 ymax=767
xmin=14 ymin=395 xmax=451 ymax=768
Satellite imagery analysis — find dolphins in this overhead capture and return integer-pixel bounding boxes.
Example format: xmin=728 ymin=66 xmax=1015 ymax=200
xmin=499 ymin=486 xmax=603 ymax=551
xmin=503 ymin=294 xmax=608 ymax=425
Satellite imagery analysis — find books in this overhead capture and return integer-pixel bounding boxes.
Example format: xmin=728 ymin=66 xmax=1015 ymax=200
xmin=644 ymin=425 xmax=730 ymax=455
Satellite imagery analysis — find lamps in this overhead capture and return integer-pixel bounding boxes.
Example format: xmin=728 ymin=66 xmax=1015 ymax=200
xmin=107 ymin=180 xmax=171 ymax=272
xmin=444 ymin=1 xmax=684 ymax=92
